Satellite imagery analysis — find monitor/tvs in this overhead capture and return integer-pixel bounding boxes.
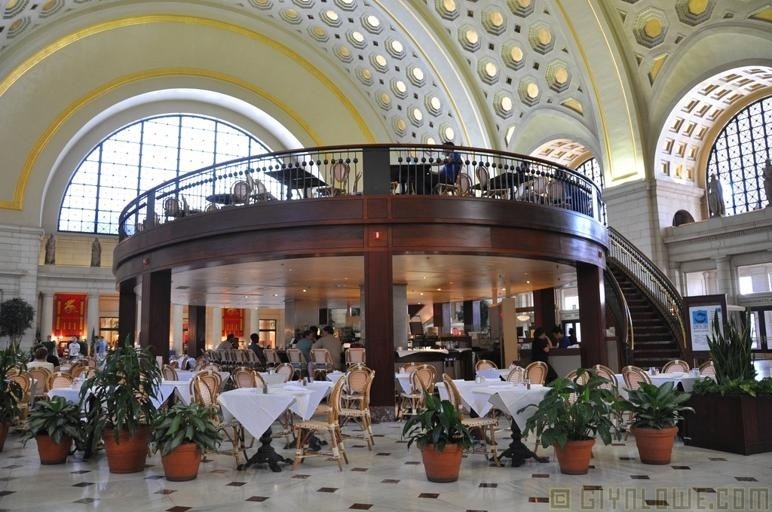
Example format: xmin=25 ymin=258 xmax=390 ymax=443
xmin=289 ymin=338 xmax=297 ymax=346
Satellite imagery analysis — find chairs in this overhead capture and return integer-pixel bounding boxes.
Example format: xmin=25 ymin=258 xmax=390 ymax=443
xmin=398 ymin=358 xmax=721 ymax=469
xmin=130 ymin=156 xmax=574 ymax=232
xmin=1 ymin=341 xmax=376 ymax=475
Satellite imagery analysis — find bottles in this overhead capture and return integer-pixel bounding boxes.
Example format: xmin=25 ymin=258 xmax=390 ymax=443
xmin=475 ymin=375 xmax=485 ymax=383
xmin=297 ymin=376 xmax=308 ymax=388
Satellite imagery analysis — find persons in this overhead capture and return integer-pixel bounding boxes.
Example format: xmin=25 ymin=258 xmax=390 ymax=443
xmin=762 ymin=158 xmax=772 ymax=207
xmin=708 ymin=173 xmax=725 ymax=216
xmin=427 ymin=141 xmax=462 ymax=184
xmin=568 ymin=328 xmax=577 ymax=345
xmin=217 ymin=325 xmax=345 ymax=370
xmin=25 ymin=334 xmax=107 ymax=370
xmin=552 ymin=326 xmax=571 ymax=352
xmin=531 ymin=327 xmax=553 ymax=361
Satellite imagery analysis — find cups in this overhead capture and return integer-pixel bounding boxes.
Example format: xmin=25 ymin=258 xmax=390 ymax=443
xmin=648 ymin=366 xmax=699 ymax=378
xmin=265 ymin=368 xmax=275 ymax=375
xmin=520 ymin=378 xmax=530 ymax=390
xmin=413 ymin=345 xmax=431 ymax=350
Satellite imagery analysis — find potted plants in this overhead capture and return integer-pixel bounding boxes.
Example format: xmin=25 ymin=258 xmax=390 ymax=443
xmin=689 ymin=311 xmax=770 ymax=456
xmin=150 ymin=398 xmax=226 ymax=481
xmin=516 ymin=367 xmax=635 ymax=477
xmin=396 ymin=386 xmax=472 ymax=485
